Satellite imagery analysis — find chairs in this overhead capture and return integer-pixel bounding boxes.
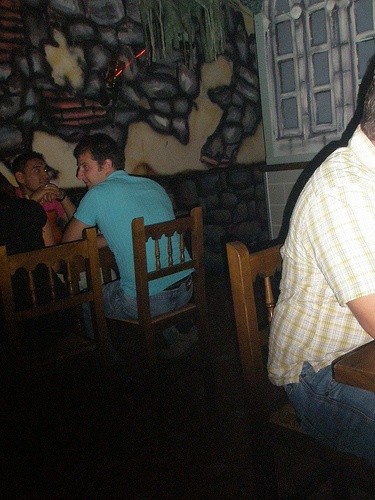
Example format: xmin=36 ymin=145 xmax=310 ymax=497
xmin=96 ymin=206 xmax=218 ymax=419
xmin=224 ymin=238 xmax=375 ymax=500
xmin=0 ymin=227 xmax=116 ymax=446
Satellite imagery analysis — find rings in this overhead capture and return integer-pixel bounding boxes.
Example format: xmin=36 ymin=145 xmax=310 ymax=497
xmin=44 ymin=184 xmax=48 ymax=188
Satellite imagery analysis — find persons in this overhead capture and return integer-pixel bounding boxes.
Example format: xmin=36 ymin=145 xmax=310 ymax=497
xmin=13 ymin=153 xmax=97 ymax=357
xmin=62 ymin=133 xmax=195 ymax=353
xmin=0 ymin=174 xmax=65 ymax=335
xmin=265 ymin=70 xmax=375 ymax=459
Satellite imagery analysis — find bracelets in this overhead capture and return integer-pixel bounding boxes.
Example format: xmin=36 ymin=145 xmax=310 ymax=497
xmin=56 ymin=194 xmax=66 ymax=202
